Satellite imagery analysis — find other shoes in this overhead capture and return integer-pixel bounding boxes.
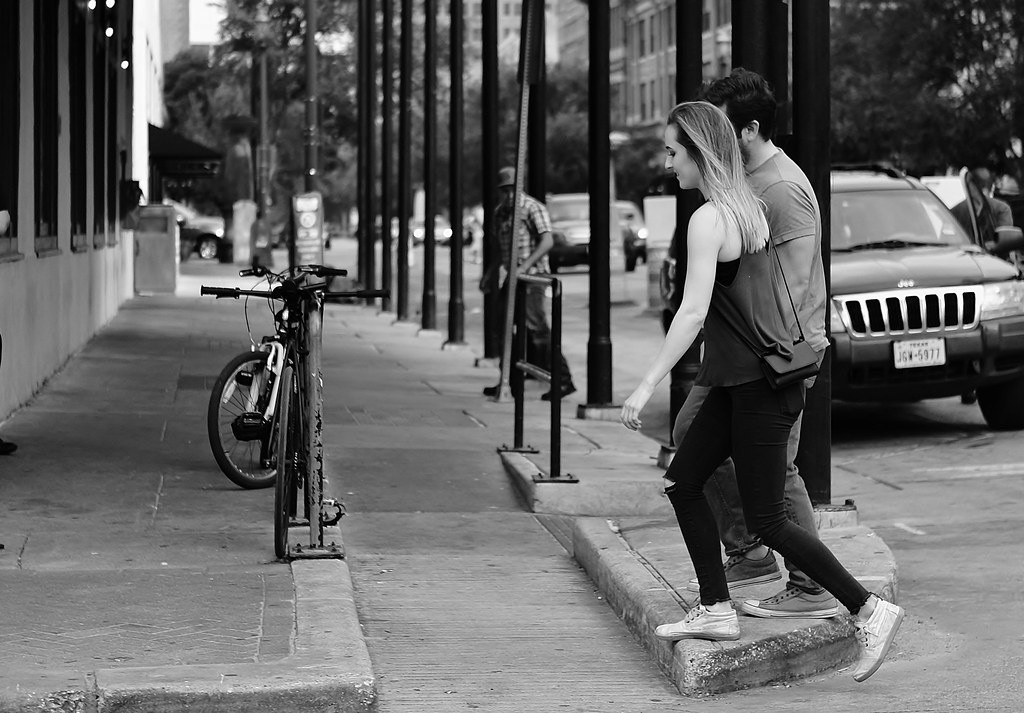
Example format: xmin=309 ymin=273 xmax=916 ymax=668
xmin=484 ymin=385 xmax=497 ymax=396
xmin=542 ymin=381 xmax=576 ymax=400
xmin=961 ymin=390 xmax=976 ymax=404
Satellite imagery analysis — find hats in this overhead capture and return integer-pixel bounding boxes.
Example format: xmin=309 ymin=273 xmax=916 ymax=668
xmin=498 ymin=167 xmax=515 ymax=187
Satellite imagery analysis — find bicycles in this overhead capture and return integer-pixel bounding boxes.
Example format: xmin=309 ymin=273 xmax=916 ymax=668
xmin=199 ymin=262 xmax=389 ymax=561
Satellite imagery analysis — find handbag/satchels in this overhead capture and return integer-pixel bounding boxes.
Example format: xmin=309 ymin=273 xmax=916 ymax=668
xmin=760 ymin=340 xmax=819 ymax=389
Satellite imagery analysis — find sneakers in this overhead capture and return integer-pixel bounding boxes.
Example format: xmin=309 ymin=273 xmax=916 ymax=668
xmin=654 ymin=604 xmax=740 ymax=641
xmin=741 ymin=586 xmax=839 ymax=618
xmin=687 ymin=548 xmax=782 ymax=591
xmin=854 ymin=599 xmax=906 ymax=682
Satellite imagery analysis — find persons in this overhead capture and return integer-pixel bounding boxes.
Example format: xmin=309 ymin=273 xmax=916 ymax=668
xmin=478 ymin=167 xmax=577 ymax=401
xmin=0 ymin=211 xmax=19 ymax=455
xmin=950 ymin=169 xmax=1014 ymax=263
xmin=672 ymin=68 xmax=837 ymax=620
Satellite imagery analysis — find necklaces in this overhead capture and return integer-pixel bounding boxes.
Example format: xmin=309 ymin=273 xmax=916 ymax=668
xmin=620 ymin=101 xmax=905 ymax=683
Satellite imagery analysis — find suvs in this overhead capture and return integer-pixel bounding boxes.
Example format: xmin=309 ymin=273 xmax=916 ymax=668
xmin=657 ymin=163 xmax=1024 ymax=430
xmin=546 ymin=193 xmax=590 ymax=273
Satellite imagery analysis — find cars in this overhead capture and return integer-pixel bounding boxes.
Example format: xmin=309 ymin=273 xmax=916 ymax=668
xmin=163 ymin=195 xmax=273 ymax=259
xmin=613 ymin=202 xmax=647 ymax=263
xmin=282 ymin=213 xmax=452 ymax=250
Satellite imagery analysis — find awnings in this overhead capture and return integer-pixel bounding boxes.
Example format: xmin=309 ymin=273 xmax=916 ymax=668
xmin=148 ymin=123 xmax=223 ymax=182
